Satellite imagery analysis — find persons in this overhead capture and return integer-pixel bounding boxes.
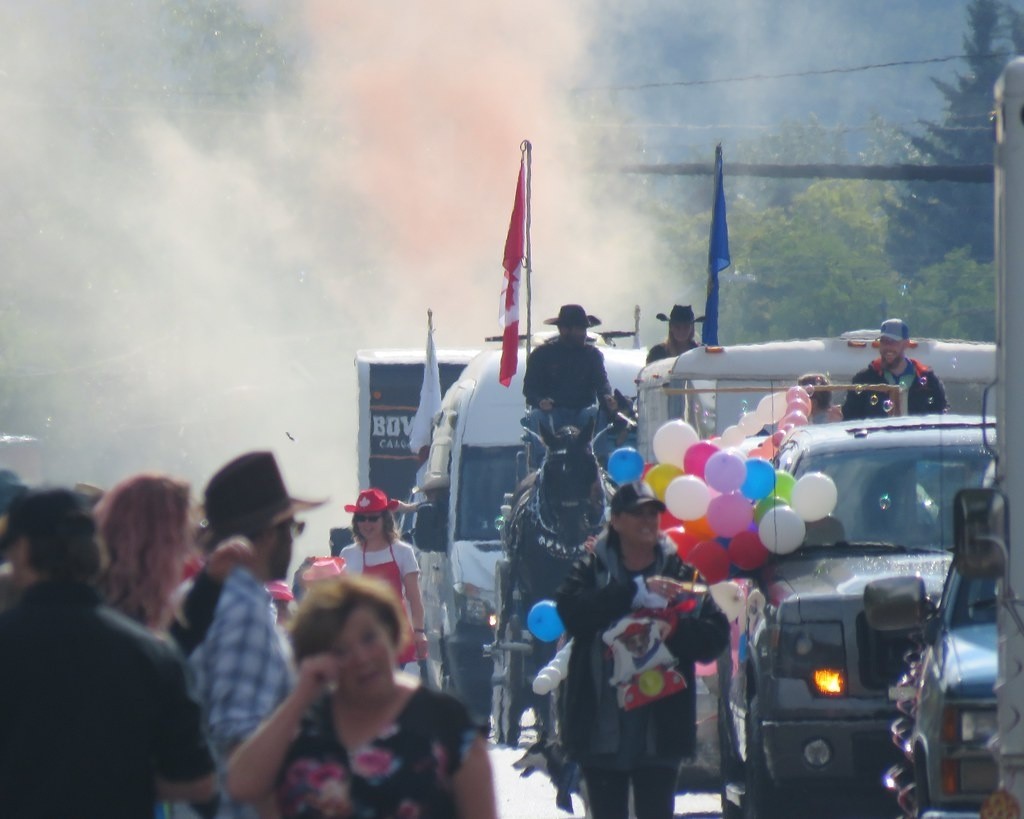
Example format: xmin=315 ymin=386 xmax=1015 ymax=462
xmin=796 ymin=373 xmax=839 ymax=424
xmin=216 ymin=572 xmax=499 ymax=819
xmin=522 ymin=303 xmax=619 ymax=471
xmin=646 ymin=304 xmax=705 ymax=365
xmin=0 ymin=465 xmax=258 ymax=819
xmin=91 ymin=474 xmax=194 ymax=632
xmin=157 ymin=451 xmax=329 ymax=819
xmin=339 ymin=488 xmax=429 ymax=669
xmin=841 ymin=318 xmax=952 ymax=420
xmin=555 ymin=480 xmax=732 ymax=819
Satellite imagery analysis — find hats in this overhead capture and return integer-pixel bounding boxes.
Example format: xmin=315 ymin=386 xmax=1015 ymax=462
xmin=411 ymin=471 xmax=448 ymax=494
xmin=656 ymin=303 xmax=708 ymax=325
xmin=544 ymin=304 xmax=601 ymax=329
xmin=344 ymin=489 xmax=400 ymax=513
xmin=198 ymin=452 xmax=328 ymax=554
xmin=611 ymin=483 xmax=667 ymax=515
xmin=877 ymin=318 xmax=909 ymax=342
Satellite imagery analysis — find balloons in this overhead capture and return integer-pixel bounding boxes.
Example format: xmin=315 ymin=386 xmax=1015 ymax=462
xmin=527 ymin=600 xmax=565 ymax=642
xmin=608 ymin=447 xmax=645 ymax=485
xmin=646 ymin=385 xmax=838 ymax=677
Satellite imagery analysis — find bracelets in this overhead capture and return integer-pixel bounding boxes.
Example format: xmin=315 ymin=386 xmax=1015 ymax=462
xmin=413 ymin=628 xmax=427 ymax=635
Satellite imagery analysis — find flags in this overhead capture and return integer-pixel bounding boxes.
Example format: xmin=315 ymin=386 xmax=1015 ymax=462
xmin=702 ymin=146 xmax=731 ymax=346
xmin=498 ymin=153 xmax=525 ymax=387
xmin=409 ymin=329 xmax=443 ymax=455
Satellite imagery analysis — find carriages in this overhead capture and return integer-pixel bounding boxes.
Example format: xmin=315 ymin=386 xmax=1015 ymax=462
xmin=480 ymin=374 xmax=645 ymax=754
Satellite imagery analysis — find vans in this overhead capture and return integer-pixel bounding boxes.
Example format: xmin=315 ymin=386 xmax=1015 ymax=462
xmin=626 ymin=338 xmax=1008 ymax=484
xmin=391 ymin=336 xmax=669 ymax=712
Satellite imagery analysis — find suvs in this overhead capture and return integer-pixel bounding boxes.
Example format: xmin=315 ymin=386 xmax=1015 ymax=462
xmin=860 ymin=454 xmax=1005 ymax=819
xmin=702 ymin=412 xmax=999 ymax=819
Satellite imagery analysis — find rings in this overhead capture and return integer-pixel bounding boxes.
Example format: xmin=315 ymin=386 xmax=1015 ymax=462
xmin=663 ymin=583 xmax=668 ymax=588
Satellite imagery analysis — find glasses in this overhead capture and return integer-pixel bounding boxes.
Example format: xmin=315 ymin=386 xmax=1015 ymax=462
xmin=282 ymin=518 xmax=306 ymax=536
xmin=357 ymin=515 xmax=382 ymax=523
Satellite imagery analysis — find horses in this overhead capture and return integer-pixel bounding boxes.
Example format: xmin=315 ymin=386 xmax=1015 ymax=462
xmin=503 ymin=415 xmax=620 ymax=744
